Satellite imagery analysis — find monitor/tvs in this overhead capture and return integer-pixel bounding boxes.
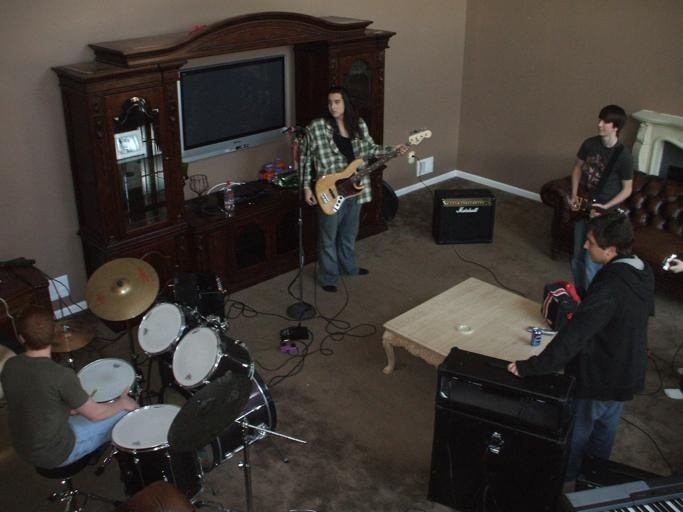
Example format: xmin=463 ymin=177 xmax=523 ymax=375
xmin=176 ymin=44 xmax=296 ymax=165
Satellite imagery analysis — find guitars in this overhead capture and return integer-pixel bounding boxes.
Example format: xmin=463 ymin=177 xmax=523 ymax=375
xmin=555 ymin=175 xmax=630 ymax=218
xmin=315 ymin=127 xmax=432 ymax=216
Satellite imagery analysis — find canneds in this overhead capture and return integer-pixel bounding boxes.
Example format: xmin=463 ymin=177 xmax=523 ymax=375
xmin=531 ymin=327 xmax=542 ymax=346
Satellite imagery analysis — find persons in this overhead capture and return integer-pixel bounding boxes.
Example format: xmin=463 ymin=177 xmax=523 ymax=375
xmin=297 ymin=87 xmax=410 ymax=292
xmin=0 ymin=305 xmax=140 ymax=469
xmin=508 ymin=210 xmax=656 ymax=495
xmin=569 ymin=104 xmax=633 ymax=299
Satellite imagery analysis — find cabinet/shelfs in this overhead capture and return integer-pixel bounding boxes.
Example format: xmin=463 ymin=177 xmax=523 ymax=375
xmin=51 ymin=12 xmax=396 ymax=332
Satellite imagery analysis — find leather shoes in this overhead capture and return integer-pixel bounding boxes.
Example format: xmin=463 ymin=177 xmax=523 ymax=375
xmin=323 ymin=286 xmax=337 ymax=292
xmin=358 ymin=268 xmax=368 ymax=275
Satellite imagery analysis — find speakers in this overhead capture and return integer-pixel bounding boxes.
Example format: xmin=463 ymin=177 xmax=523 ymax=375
xmin=431 ymin=188 xmax=497 ymax=246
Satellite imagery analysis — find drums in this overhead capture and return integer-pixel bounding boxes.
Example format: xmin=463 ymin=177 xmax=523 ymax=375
xmin=77 ymin=359 xmax=145 ymax=412
xmin=191 ymin=368 xmax=276 ymax=472
xmin=109 ymin=405 xmax=205 ymax=506
xmin=138 ymin=302 xmax=207 ymax=366
xmin=171 ymin=325 xmax=253 ymax=405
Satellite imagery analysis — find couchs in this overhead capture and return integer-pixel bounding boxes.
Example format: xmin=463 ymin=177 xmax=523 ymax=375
xmin=540 ymin=169 xmax=683 ymax=303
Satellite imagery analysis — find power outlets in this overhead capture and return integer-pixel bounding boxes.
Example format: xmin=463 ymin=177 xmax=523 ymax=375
xmin=408 ymin=149 xmax=416 ymax=164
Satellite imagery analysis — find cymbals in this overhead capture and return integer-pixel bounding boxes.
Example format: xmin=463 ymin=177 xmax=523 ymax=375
xmin=51 ymin=319 xmax=96 ymax=352
xmin=87 ymin=258 xmax=160 ymax=322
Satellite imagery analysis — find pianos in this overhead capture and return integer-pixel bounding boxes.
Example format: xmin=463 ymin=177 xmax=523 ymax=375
xmin=563 ymin=479 xmax=683 ymax=512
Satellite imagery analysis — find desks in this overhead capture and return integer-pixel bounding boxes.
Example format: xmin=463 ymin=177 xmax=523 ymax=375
xmin=382 ymin=276 xmax=561 ymax=376
xmin=1 ymin=257 xmax=57 ymax=355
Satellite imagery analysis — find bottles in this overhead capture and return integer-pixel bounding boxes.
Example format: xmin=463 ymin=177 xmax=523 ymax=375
xmin=224 ymin=180 xmax=234 ymax=211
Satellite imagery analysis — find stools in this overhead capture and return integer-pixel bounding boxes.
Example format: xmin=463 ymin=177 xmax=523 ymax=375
xmin=35 ymin=454 xmax=123 ymax=511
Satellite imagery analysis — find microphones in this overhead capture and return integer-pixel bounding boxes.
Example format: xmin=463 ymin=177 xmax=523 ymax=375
xmin=283 ymin=126 xmax=306 ymax=134
